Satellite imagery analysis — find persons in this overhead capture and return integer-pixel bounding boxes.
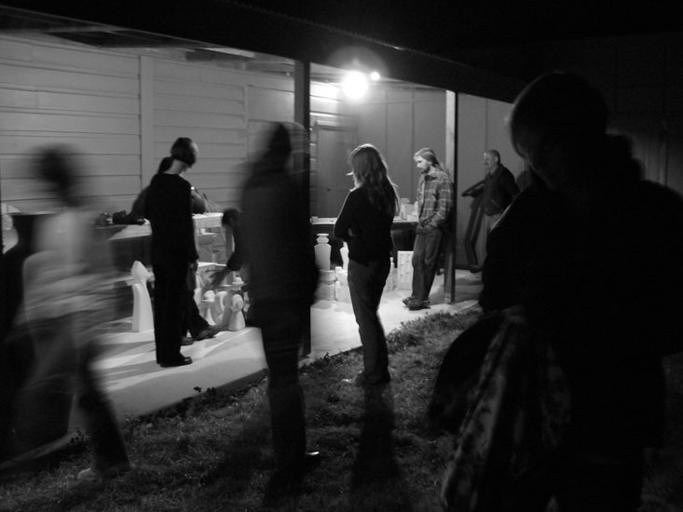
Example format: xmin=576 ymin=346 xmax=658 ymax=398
xmin=474 ymin=149 xmax=517 ymax=270
xmin=130 ymin=135 xmax=223 ymax=374
xmin=210 ymin=202 xmax=244 ymax=289
xmin=330 ymin=144 xmax=398 ymax=390
xmin=403 ymin=147 xmax=456 ymax=311
xmin=237 ymin=119 xmax=321 ymax=469
xmin=151 ymin=155 xmax=223 ymax=344
xmin=30 ymin=142 xmax=126 ymax=480
xmin=480 ymin=71 xmax=682 ymax=512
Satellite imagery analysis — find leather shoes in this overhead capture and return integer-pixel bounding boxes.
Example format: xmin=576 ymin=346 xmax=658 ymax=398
xmin=180 ymin=337 xmax=192 ymax=345
xmin=161 ymin=356 xmax=192 ymax=367
xmin=196 ymin=322 xmax=227 ymax=340
